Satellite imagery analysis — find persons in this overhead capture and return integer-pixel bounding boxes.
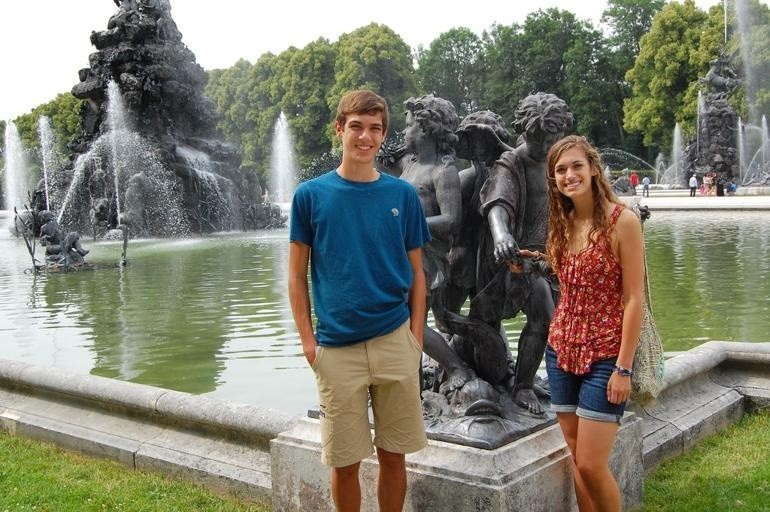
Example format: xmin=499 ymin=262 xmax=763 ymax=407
xmin=510 ymin=133 xmax=646 ymax=512
xmin=287 ymin=89 xmax=429 ymax=511
xmin=699 ymin=171 xmax=738 ymax=196
xmin=37 ymin=210 xmax=90 ymax=256
xmin=719 ymin=48 xmax=740 ymax=79
xmin=688 ymin=174 xmax=698 ymax=197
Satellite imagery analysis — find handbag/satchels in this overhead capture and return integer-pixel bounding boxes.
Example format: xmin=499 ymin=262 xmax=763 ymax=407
xmin=631 ymin=302 xmax=664 ymax=400
